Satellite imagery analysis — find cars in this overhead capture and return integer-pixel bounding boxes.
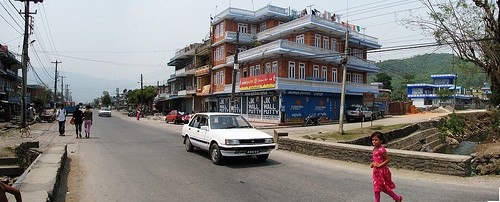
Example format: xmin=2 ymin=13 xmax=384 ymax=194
xmin=165 ymin=109 xmax=190 ymax=124
xmin=181 ymin=112 xmax=276 ymax=166
xmin=98 ymin=107 xmax=112 ymax=117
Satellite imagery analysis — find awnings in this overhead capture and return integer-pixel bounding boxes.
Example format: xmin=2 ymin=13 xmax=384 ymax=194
xmin=160 ymin=96 xmax=193 ymax=101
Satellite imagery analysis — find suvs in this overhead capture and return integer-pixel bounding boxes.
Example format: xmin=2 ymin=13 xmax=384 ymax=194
xmin=345 ymin=104 xmax=373 ymax=122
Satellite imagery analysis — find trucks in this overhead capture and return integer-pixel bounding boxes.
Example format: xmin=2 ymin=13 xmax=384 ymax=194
xmin=64 ymin=102 xmax=76 ymax=115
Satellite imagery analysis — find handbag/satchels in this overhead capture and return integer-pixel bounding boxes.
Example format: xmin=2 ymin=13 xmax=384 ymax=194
xmin=70 ymin=118 xmax=75 ymax=124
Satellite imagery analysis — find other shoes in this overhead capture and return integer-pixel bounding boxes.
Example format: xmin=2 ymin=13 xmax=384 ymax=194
xmin=395 ymin=194 xmax=403 ymax=202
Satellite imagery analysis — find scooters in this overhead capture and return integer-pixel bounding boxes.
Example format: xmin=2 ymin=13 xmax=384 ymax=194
xmin=35 ymin=109 xmax=56 ymax=123
xmin=302 ymin=112 xmax=322 ymax=127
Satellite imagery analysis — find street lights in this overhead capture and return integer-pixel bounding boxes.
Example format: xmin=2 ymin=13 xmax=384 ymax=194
xmin=226 ymin=51 xmax=238 ymax=113
xmin=137 ymin=80 xmax=144 ymax=117
xmin=54 ymin=79 xmax=60 ymax=109
xmin=22 ymin=40 xmax=36 ymax=128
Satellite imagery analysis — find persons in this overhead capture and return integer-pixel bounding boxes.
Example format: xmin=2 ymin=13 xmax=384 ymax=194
xmin=56 ymin=104 xmax=66 ymax=136
xmin=370 ymin=132 xmax=403 ymax=202
xmin=136 ymin=108 xmax=140 ymax=120
xmin=185 ymin=113 xmax=190 ymax=124
xmin=191 ymin=111 xmax=195 ymax=118
xmin=83 ymin=106 xmax=93 ymax=138
xmin=73 ymin=105 xmax=83 ymax=138
